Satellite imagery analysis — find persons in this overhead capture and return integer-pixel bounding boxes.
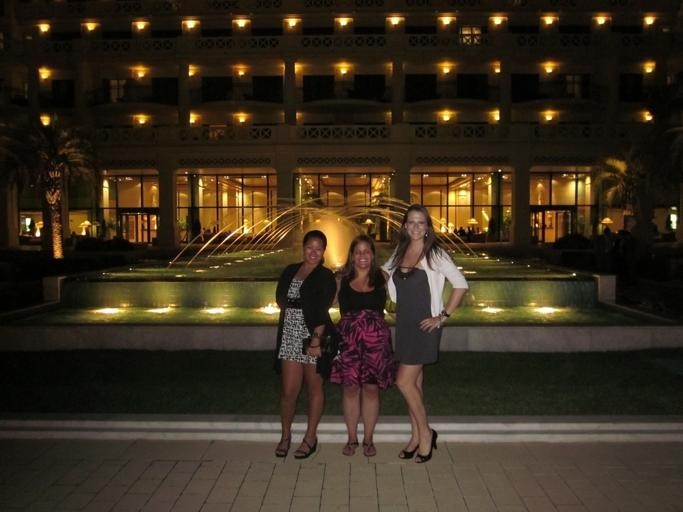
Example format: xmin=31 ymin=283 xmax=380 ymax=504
xmin=275 ymin=230 xmax=336 ymax=459
xmin=381 ymin=204 xmax=469 ymax=462
xmin=328 ymin=235 xmax=398 ymax=456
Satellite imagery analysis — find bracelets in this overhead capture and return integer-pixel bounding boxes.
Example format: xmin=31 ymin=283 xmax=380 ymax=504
xmin=438 ymin=314 xmax=441 ymax=328
xmin=312 ymin=333 xmax=322 ymax=338
xmin=308 ymin=345 xmax=320 ymax=348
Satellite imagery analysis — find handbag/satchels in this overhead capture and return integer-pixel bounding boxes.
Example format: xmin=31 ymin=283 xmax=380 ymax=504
xmin=302 ymin=335 xmax=334 ymax=355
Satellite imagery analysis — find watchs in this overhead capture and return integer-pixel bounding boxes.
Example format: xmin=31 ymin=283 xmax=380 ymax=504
xmin=442 ymin=310 xmax=450 ymax=317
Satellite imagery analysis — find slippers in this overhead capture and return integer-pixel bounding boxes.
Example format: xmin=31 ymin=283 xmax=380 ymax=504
xmin=343 ymin=442 xmax=376 ymax=456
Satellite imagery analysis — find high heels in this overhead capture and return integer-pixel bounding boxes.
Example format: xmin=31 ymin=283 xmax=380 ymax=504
xmin=294 ymin=436 xmax=317 ymax=459
xmin=275 ymin=437 xmax=291 ymax=457
xmin=398 ymin=429 xmax=438 ymax=463
